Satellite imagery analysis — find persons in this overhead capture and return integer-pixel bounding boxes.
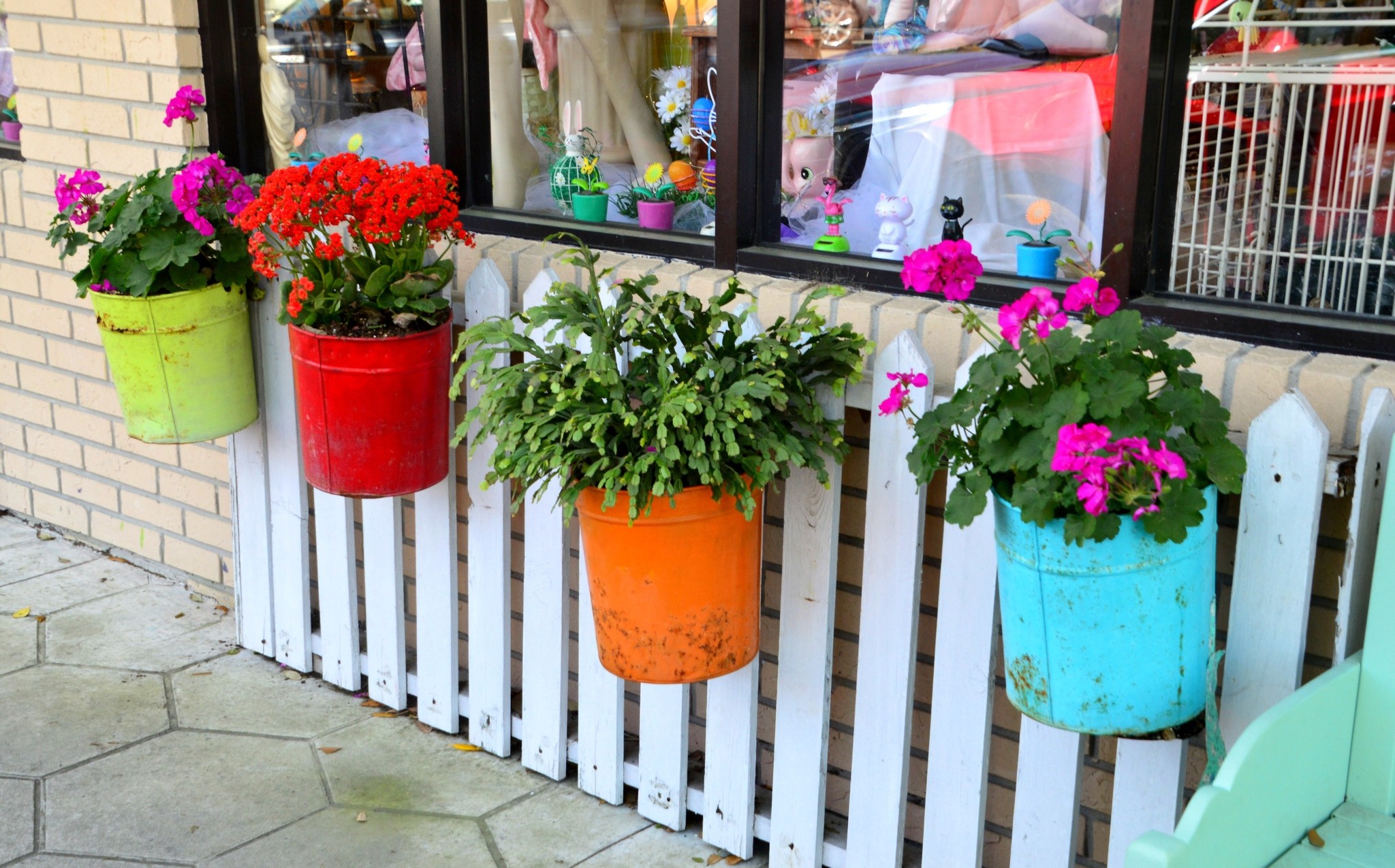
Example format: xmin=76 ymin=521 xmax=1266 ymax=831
xmin=258 ymin=34 xmax=304 ymax=170
xmin=782 ymin=109 xmax=835 ymax=217
xmin=487 ymin=0 xmax=673 ymax=211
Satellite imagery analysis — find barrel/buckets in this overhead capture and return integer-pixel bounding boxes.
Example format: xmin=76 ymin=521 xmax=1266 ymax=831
xmin=285 ymin=308 xmax=457 ymax=500
xmin=86 ymin=278 xmax=259 ymax=445
xmin=992 ymin=479 xmax=1218 ymax=736
xmin=574 ymin=464 xmax=765 ymax=683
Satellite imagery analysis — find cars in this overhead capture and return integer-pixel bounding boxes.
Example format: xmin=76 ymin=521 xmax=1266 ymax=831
xmin=774 ymin=0 xmax=1110 ymax=269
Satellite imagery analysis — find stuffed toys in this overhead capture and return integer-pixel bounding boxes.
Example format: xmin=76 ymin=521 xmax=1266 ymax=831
xmin=818 ymin=0 xmax=869 ymax=50
xmin=863 ymin=0 xmax=890 ymax=28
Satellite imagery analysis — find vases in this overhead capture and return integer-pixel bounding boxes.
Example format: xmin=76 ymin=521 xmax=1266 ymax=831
xmin=2 ymin=122 xmax=23 ymax=140
xmin=292 ymin=159 xmax=318 ymax=174
xmin=638 ymin=201 xmax=674 ymax=230
xmin=571 ymin=194 xmax=608 ymax=222
xmin=1014 ymin=241 xmax=1060 ymax=278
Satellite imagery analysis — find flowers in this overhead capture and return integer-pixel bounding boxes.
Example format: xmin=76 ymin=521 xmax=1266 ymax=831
xmin=572 ymin=156 xmax=608 ymax=195
xmin=874 ymin=237 xmax=1245 ymax=552
xmin=48 ymin=84 xmax=267 ymax=314
xmin=235 ymin=147 xmax=475 ymax=331
xmin=631 ymin=161 xmax=676 ymax=198
xmin=1005 ymin=196 xmax=1071 ymax=242
xmin=2 ymin=92 xmax=18 ymax=122
xmin=348 ymin=135 xmax=379 ymax=160
xmin=290 ymin=121 xmax=327 ymax=160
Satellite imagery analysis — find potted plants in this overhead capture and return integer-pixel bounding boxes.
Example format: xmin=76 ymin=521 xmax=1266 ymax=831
xmin=451 ymin=225 xmax=874 ymax=681
